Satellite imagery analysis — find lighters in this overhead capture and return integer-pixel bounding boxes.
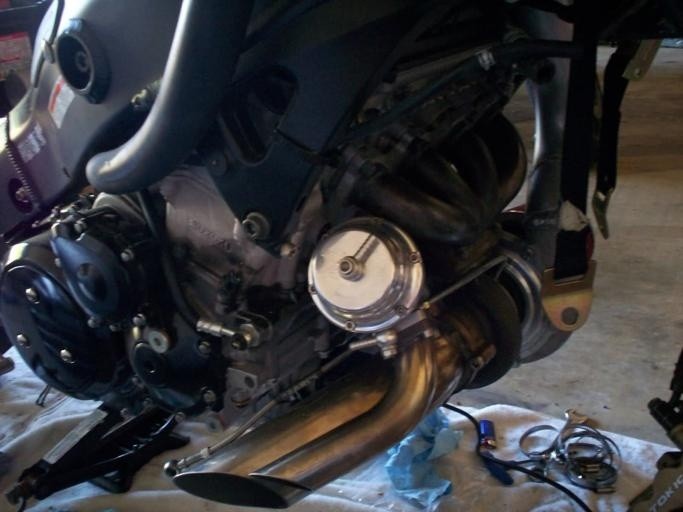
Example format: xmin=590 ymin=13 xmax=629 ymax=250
xmin=479 ymin=420 xmax=496 ymax=448
